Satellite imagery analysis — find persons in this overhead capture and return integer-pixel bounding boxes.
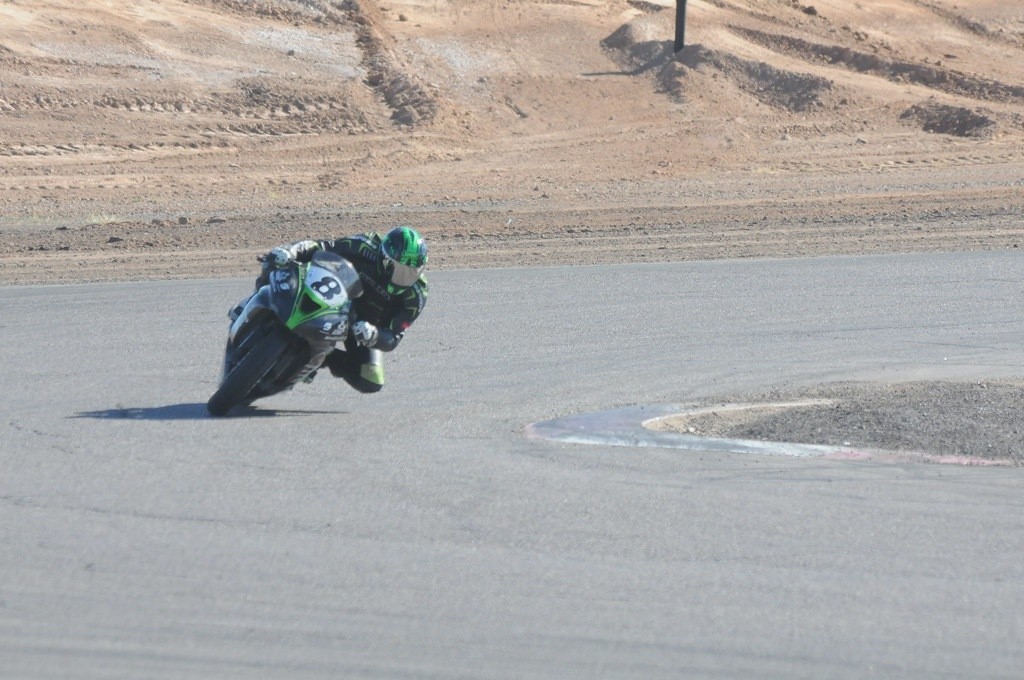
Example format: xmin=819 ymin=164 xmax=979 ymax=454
xmin=227 ymin=226 xmax=430 ymax=394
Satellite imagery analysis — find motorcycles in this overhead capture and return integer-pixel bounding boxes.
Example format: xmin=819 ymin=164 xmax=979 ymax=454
xmin=206 ymin=249 xmax=364 ymax=418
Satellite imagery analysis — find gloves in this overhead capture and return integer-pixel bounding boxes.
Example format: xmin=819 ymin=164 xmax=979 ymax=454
xmin=355 ymin=321 xmax=378 ymax=349
xmin=268 ymin=245 xmax=291 ymax=269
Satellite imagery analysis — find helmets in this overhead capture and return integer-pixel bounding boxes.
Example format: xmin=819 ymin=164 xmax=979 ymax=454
xmin=381 ymin=227 xmax=429 ymax=296
